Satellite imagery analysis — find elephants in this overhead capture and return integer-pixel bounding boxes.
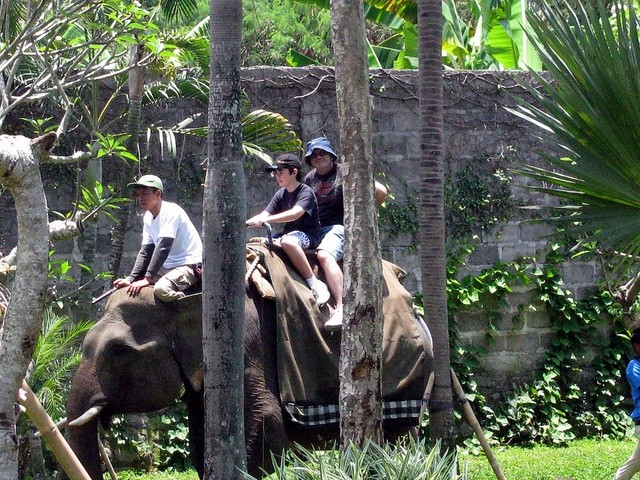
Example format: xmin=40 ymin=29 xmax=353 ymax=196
xmin=30 ymin=236 xmax=434 ymax=479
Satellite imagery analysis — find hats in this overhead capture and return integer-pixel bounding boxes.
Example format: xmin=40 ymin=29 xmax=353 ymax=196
xmin=264 ymin=153 xmax=303 ymax=175
xmin=305 ymin=137 xmax=338 ymax=167
xmin=126 ymin=174 xmax=164 ymax=194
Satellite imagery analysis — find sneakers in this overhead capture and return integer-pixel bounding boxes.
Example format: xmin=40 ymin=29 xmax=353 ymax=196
xmin=310 ymin=279 xmax=331 ymax=308
xmin=324 ymin=302 xmax=343 ymax=332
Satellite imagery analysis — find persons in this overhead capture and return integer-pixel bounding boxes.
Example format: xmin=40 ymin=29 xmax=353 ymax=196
xmin=612 ymin=330 xmax=640 ymax=480
xmin=113 ymin=175 xmax=203 ymax=302
xmin=246 ymin=153 xmax=331 ymax=309
xmin=302 ymin=136 xmax=388 ymax=331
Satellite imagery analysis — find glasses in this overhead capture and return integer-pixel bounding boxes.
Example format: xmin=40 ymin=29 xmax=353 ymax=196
xmin=310 ymin=150 xmax=330 ymax=159
